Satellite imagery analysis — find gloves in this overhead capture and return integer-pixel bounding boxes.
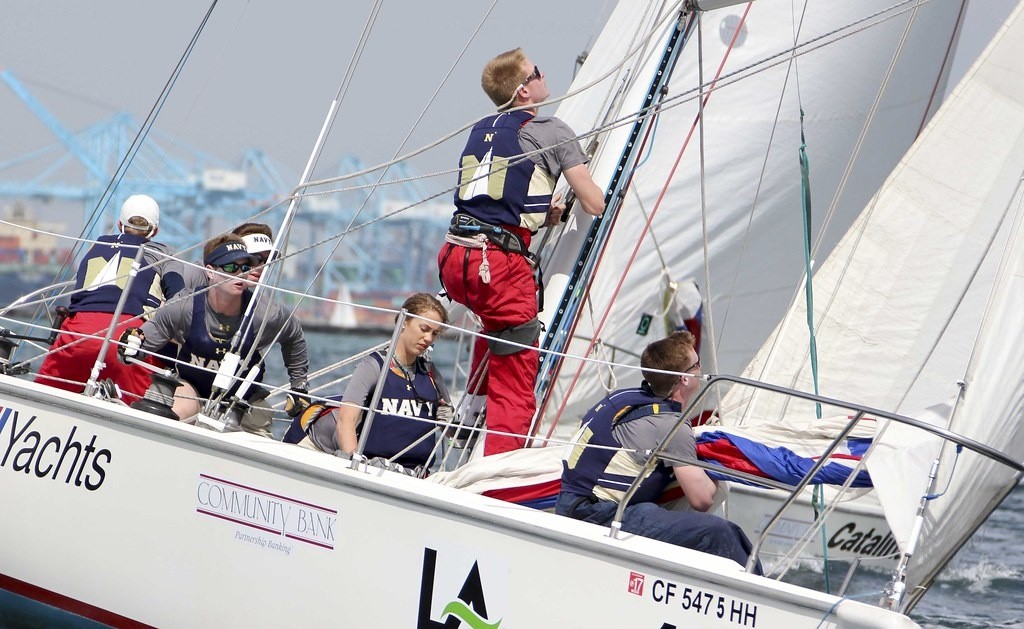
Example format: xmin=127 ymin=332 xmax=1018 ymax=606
xmin=284 ymin=387 xmax=311 ymax=418
xmin=117 ymin=326 xmax=146 ymax=358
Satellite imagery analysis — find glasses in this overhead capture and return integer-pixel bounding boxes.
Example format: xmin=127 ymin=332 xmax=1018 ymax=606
xmin=684 ymin=356 xmax=701 ymax=374
xmin=211 ymin=262 xmax=251 ymax=273
xmin=252 ymin=253 xmax=267 ymax=264
xmin=522 ymin=65 xmax=540 ymax=86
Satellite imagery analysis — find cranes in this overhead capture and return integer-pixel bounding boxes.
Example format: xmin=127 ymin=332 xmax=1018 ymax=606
xmin=0 ymin=71 xmax=452 ymax=295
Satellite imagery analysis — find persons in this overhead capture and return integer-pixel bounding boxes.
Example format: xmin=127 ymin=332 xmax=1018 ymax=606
xmin=182 ymin=223 xmax=282 ymax=287
xmin=436 ymin=47 xmax=605 ymax=456
xmin=337 ymin=293 xmax=460 ymax=479
xmin=117 ymin=233 xmax=311 ymax=439
xmin=555 ymin=331 xmax=764 ymax=576
xmin=34 ymin=195 xmax=185 ymax=405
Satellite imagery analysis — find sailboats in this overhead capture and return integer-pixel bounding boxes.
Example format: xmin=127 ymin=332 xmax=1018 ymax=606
xmin=0 ymin=0 xmax=1023 ymax=628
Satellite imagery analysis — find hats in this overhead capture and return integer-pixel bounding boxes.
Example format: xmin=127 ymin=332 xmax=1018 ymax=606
xmin=204 ymin=241 xmax=258 ymax=268
xmin=240 ymin=233 xmax=281 ymax=259
xmin=120 ymin=195 xmax=159 ymax=231
